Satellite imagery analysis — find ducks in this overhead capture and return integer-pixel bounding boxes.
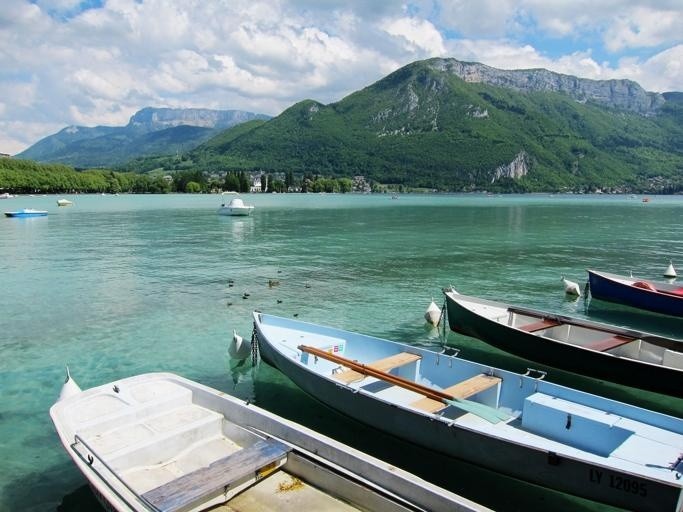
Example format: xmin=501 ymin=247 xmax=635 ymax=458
xmin=269 ymin=280 xmax=280 ymax=285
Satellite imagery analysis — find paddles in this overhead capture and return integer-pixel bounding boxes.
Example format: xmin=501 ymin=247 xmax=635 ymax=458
xmin=298 ymin=345 xmax=510 ymax=425
xmin=508 ymin=307 xmax=683 ymax=353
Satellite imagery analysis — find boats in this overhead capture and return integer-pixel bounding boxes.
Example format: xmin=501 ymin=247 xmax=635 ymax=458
xmin=0 ymin=192 xmax=15 ymax=199
xmin=2 ymin=208 xmax=49 ymax=218
xmin=56 ymin=198 xmax=73 ymax=207
xmin=582 ymin=267 xmax=682 ymax=320
xmin=47 ymin=366 xmax=497 ymax=512
xmin=249 ymin=309 xmax=682 ymax=512
xmin=442 ymin=286 xmax=682 ymax=414
xmin=216 ymin=190 xmax=255 ymax=216
